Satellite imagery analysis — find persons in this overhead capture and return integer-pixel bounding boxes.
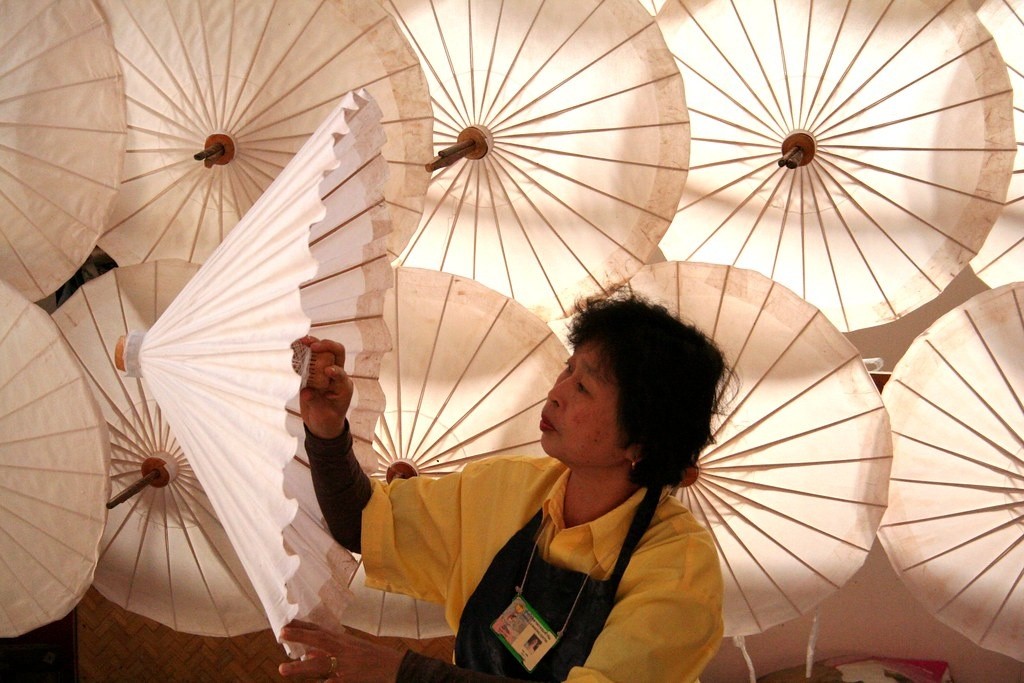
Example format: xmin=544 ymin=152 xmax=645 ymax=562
xmin=525 ymin=638 xmax=538 ymax=653
xmin=279 ymin=298 xmax=723 ymax=683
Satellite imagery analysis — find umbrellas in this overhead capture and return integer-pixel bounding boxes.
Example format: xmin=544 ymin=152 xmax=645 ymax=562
xmin=644 ymin=0 xmax=1017 ymax=332
xmin=548 ymin=263 xmax=893 ymax=638
xmin=0 ymin=0 xmax=688 ymax=683
xmin=932 ymin=0 xmax=1024 ymax=289
xmin=878 ymin=281 xmax=1024 ymax=661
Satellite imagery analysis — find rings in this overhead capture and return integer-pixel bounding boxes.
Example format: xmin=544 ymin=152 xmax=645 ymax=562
xmin=329 ymin=656 xmax=337 ymax=673
